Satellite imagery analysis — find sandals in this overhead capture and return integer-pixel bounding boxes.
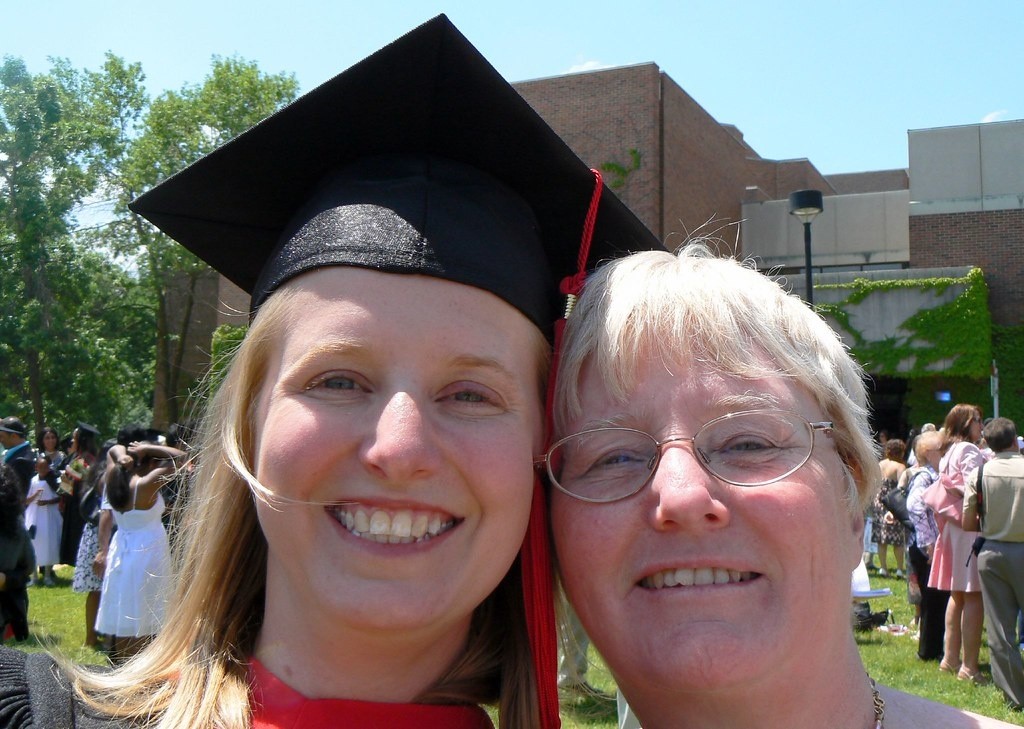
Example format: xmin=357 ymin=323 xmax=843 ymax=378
xmin=939 ymin=659 xmax=962 ymax=673
xmin=957 ymin=667 xmax=990 ymax=685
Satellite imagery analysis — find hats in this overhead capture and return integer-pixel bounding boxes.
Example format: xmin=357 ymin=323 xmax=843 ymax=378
xmin=127 ymin=12 xmax=674 ymax=728
xmin=76 ymin=420 xmax=103 ymax=437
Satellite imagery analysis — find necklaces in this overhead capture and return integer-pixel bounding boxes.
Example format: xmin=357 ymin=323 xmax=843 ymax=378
xmin=866 ymin=672 xmax=885 ymax=729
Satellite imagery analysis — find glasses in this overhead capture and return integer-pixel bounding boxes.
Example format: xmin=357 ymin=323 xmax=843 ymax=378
xmin=973 ymin=417 xmax=980 ymax=423
xmin=534 ymin=410 xmax=834 ymax=503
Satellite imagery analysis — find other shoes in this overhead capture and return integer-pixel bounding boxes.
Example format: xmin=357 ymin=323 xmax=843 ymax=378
xmin=28 ymin=578 xmax=39 ymax=585
xmin=44 ymin=577 xmax=55 ymax=586
xmin=879 ymin=567 xmax=887 ymax=575
xmin=897 ymin=569 xmax=902 ymax=577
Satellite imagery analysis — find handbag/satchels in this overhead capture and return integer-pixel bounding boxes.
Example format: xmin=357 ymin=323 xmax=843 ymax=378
xmin=881 ymin=470 xmax=934 ymax=533
xmin=920 ymin=444 xmax=964 ymax=529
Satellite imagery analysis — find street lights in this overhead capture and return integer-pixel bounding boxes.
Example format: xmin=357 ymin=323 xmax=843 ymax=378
xmin=786 ymin=187 xmax=824 ymax=311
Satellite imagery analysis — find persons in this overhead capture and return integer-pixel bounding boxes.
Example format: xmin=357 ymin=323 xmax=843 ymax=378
xmin=1 ymin=14 xmax=672 ymax=728
xmin=0 ymin=415 xmax=200 ymax=666
xmin=534 ymin=212 xmax=1024 ymax=729
xmin=863 ymin=403 xmax=1023 ymax=711
